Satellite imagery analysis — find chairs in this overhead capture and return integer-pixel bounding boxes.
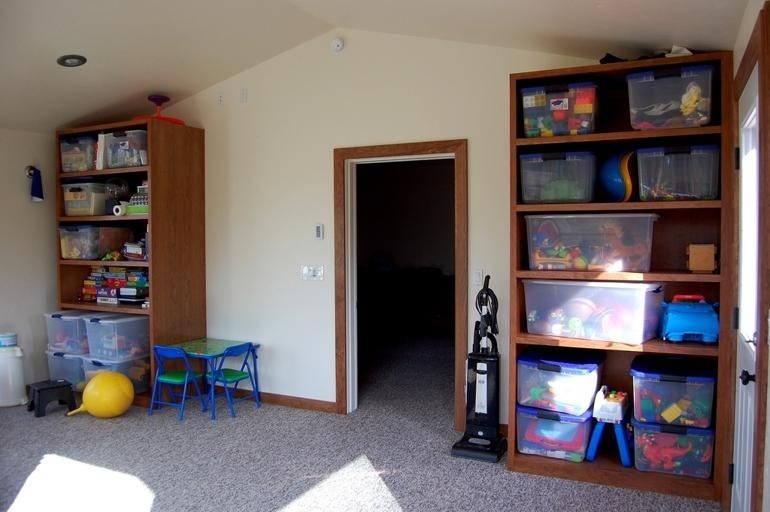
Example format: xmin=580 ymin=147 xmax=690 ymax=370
xmin=203 ymin=343 xmax=261 ymax=419
xmin=152 ymin=346 xmax=205 ymax=418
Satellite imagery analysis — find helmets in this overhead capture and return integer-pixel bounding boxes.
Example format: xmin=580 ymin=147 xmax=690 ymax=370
xmin=598 ymin=150 xmax=642 ymax=199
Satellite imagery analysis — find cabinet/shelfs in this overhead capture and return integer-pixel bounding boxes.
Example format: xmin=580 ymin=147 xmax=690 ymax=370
xmin=57 ymin=118 xmax=207 ymax=408
xmin=507 ymin=50 xmax=734 ymax=501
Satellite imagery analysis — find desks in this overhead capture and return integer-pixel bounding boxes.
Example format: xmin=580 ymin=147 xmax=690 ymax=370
xmin=159 ymin=337 xmax=259 ymax=416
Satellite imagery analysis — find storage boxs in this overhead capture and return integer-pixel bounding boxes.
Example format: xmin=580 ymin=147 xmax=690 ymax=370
xmin=80 ymin=360 xmax=148 ymax=391
xmin=629 ymin=69 xmax=711 ymax=130
xmin=629 ymin=355 xmax=715 ymax=427
xmin=45 ymin=358 xmax=85 ymax=389
xmin=639 ymin=146 xmax=719 ymax=199
xmin=521 ymin=283 xmax=664 ymax=346
xmin=85 ymin=316 xmax=148 ymax=360
xmin=524 ymin=82 xmax=596 ymax=137
xmin=516 ymin=408 xmax=594 ymax=462
xmin=523 ymin=151 xmax=595 ymax=203
xmin=516 ymin=353 xmax=600 ymax=413
xmin=525 ymin=212 xmax=660 ymax=272
xmin=633 ymin=421 xmax=715 ymax=478
xmin=42 ymin=311 xmax=86 ymax=354
